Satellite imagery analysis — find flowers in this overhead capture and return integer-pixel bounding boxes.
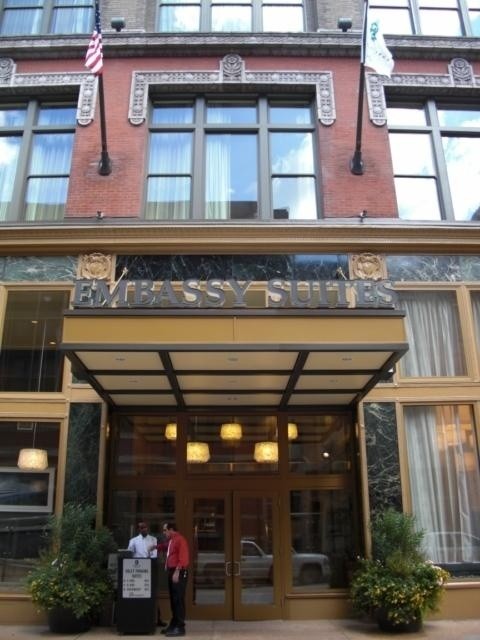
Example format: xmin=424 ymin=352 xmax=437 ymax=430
xmin=349 ymin=505 xmax=450 ymax=625
xmin=22 ymin=500 xmax=121 ymax=620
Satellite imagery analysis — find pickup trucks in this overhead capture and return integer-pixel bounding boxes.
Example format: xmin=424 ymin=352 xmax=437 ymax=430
xmin=196 ymin=539 xmax=272 ymax=581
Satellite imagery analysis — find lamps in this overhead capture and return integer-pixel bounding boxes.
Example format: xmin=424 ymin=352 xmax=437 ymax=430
xmin=15 ymin=320 xmax=49 ymax=473
xmin=162 ymin=413 xmax=300 ymax=466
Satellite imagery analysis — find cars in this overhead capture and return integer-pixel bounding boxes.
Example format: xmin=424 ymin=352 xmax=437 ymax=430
xmin=419 ymin=531 xmax=480 ymax=576
xmin=290 ymin=546 xmax=330 ymax=584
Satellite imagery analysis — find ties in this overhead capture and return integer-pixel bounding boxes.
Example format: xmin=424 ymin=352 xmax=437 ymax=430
xmin=165 ymin=538 xmax=172 ymax=571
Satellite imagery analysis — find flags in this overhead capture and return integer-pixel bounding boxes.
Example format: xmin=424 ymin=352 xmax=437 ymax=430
xmin=83 ymin=6 xmax=104 ymax=78
xmin=361 ymin=5 xmax=395 ymax=78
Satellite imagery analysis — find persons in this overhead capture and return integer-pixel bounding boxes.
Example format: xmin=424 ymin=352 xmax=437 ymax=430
xmin=147 ymin=522 xmax=189 ymax=636
xmin=128 ymin=521 xmax=157 ymax=558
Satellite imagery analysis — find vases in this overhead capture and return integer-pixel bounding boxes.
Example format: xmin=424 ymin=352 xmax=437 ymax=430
xmin=44 ymin=604 xmax=98 ymax=634
xmin=371 ymin=602 xmax=423 ymax=634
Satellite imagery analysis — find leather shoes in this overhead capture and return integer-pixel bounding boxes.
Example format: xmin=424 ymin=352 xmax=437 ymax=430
xmin=157 ymin=621 xmax=185 ymax=636
